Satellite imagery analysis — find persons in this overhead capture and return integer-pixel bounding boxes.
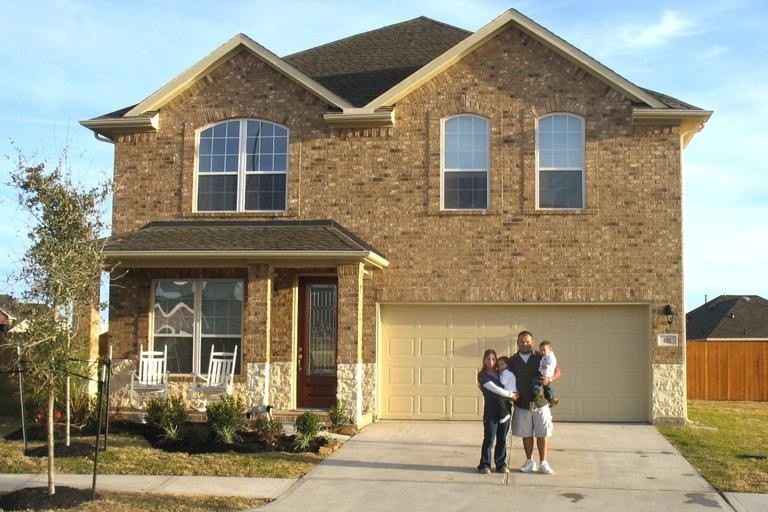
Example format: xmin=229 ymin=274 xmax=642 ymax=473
xmin=478 ymin=330 xmax=562 ymax=474
xmin=495 ymin=356 xmax=518 ymax=424
xmin=530 ymin=340 xmax=560 ymax=407
xmin=477 ymin=349 xmax=520 ymax=474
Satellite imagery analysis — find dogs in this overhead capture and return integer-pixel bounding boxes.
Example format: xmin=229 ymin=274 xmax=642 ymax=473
xmin=244 ymin=404 xmax=275 ymax=422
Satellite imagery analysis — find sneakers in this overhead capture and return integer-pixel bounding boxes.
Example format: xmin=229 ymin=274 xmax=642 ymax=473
xmin=539 ymin=462 xmax=552 ymax=473
xmin=549 ymin=398 xmax=559 ymax=408
xmin=496 ymin=468 xmax=509 ymax=472
xmin=520 ymin=462 xmax=536 ymax=472
xmin=532 ymin=392 xmax=543 ymax=402
xmin=499 ymin=414 xmax=510 ymax=424
xmin=479 ymin=468 xmax=490 ymax=474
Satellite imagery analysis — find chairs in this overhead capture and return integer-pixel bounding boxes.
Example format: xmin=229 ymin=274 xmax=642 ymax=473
xmin=129 ymin=343 xmax=238 ymax=414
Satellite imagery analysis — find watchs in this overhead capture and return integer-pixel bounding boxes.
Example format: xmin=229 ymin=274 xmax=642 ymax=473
xmin=549 ymin=377 xmax=553 ymax=384
